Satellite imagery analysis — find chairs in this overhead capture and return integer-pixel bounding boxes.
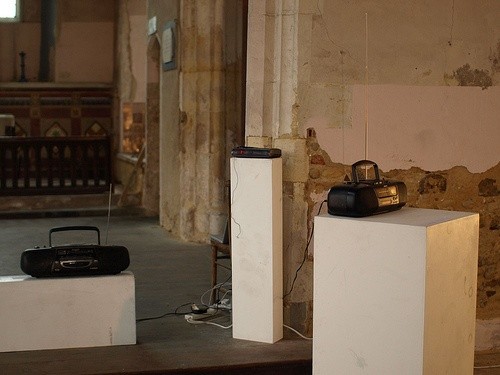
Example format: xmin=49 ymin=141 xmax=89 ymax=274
xmin=209 ymin=179 xmax=231 ymax=305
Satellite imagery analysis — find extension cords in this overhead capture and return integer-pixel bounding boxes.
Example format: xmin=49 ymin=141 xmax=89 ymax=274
xmin=213 ymin=298 xmax=231 ymax=311
xmin=183 ymin=308 xmax=220 ymax=320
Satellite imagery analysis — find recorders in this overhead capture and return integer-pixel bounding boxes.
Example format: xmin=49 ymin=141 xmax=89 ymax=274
xmin=20 ymin=225 xmax=129 ymax=276
xmin=327 ymin=159 xmax=406 ymax=216
